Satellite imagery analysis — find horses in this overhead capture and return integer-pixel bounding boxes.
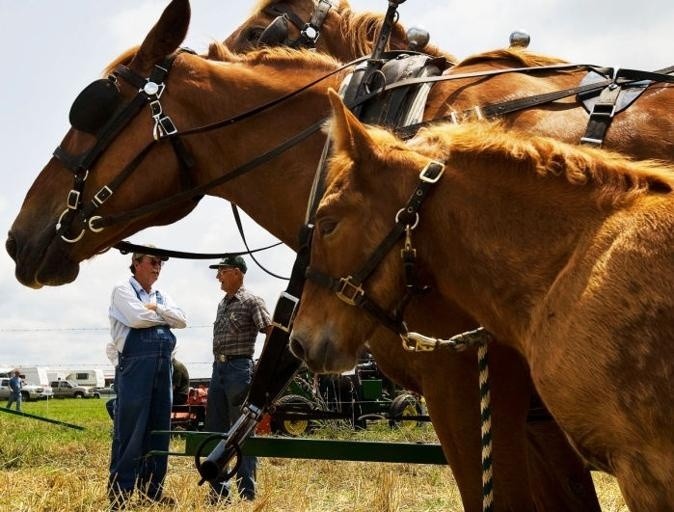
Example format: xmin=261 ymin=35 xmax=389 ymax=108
xmin=282 ymin=84 xmax=674 ymax=511
xmin=201 ymin=0 xmax=462 ymax=79
xmin=4 ymin=0 xmax=674 ymax=509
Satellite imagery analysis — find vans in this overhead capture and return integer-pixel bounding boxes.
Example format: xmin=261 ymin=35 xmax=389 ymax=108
xmin=67 ymin=370 xmax=105 ymax=387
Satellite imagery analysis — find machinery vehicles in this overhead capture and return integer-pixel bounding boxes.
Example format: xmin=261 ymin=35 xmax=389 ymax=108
xmin=169 ymin=351 xmax=425 ymax=431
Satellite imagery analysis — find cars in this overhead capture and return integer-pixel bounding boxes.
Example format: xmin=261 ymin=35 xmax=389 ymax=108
xmin=50 ymin=380 xmax=93 ymax=398
xmin=91 ymin=383 xmax=114 ymax=398
xmin=0 ymin=377 xmax=54 ymax=401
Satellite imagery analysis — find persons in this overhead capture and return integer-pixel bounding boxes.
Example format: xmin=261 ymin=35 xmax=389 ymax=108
xmin=106 ymin=243 xmax=192 ymax=509
xmin=203 ymin=252 xmax=275 ymax=506
xmin=5 ymin=369 xmax=27 ymax=412
xmin=167 ymin=355 xmax=191 ymax=407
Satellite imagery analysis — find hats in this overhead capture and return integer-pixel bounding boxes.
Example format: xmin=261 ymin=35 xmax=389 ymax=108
xmin=209 ymin=255 xmax=248 ymax=274
xmin=132 ymin=243 xmax=169 ymax=261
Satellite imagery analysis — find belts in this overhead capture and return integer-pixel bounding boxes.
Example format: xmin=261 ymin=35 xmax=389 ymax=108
xmin=214 ymin=354 xmax=252 ymax=364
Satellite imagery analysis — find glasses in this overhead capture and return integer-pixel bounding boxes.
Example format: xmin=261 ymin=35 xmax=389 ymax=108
xmin=151 ymin=260 xmax=162 ymax=265
